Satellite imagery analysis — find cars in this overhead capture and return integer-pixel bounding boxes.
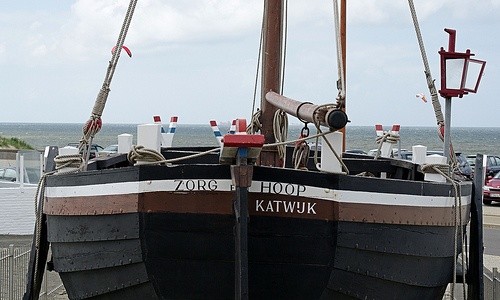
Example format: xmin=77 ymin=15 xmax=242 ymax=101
xmin=98 ymin=145 xmax=117 ymax=156
xmin=482 ymin=170 xmax=500 ymax=205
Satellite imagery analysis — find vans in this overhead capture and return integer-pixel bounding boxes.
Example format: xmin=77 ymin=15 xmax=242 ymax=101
xmin=465 ymin=154 xmax=500 ymax=178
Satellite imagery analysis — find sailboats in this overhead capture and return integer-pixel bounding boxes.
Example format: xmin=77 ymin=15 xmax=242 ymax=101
xmin=22 ymin=0 xmax=486 ymax=300
